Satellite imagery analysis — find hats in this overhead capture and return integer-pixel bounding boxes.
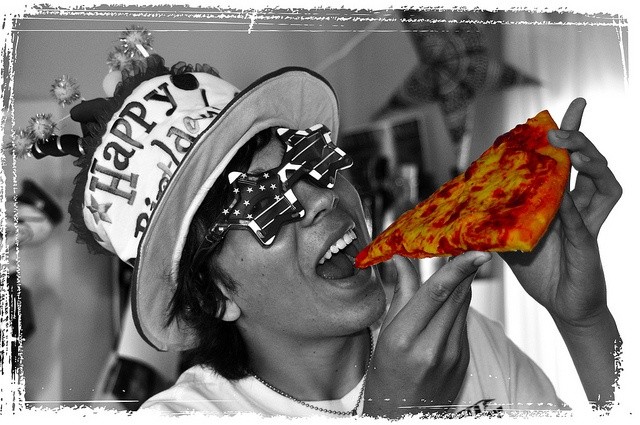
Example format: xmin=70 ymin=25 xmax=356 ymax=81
xmin=68 ymin=62 xmax=341 ymax=354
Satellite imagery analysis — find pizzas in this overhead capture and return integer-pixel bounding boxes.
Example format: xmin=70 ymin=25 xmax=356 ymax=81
xmin=355 ymin=109 xmax=570 ymax=270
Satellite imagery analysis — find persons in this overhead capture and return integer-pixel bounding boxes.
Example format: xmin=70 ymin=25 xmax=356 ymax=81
xmin=8 ymin=26 xmax=623 ymax=418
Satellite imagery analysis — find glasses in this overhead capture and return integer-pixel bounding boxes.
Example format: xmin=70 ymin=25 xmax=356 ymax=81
xmin=189 ymin=123 xmax=354 ymax=276
